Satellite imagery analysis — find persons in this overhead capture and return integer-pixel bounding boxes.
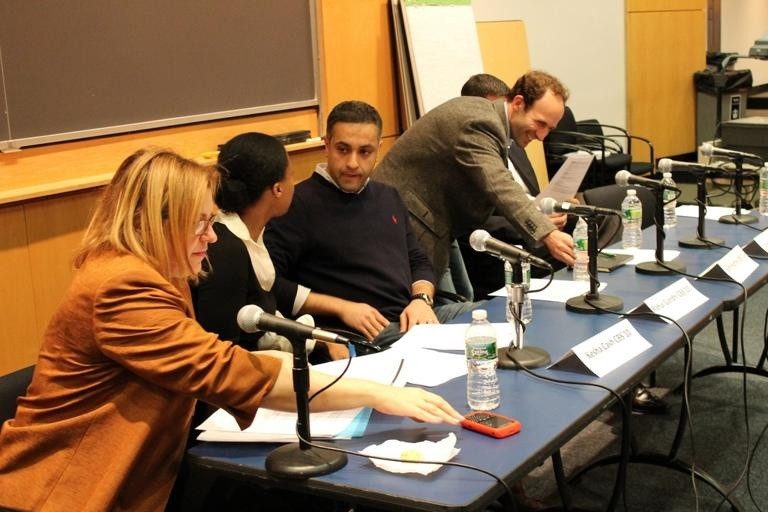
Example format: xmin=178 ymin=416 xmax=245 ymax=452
xmin=1 ymin=145 xmax=466 ymax=511
xmin=189 ymin=132 xmax=351 ymax=429
xmin=268 ymin=100 xmax=485 ymax=353
xmin=461 ymin=73 xmax=667 ymax=411
xmin=371 ymin=70 xmax=575 ymax=303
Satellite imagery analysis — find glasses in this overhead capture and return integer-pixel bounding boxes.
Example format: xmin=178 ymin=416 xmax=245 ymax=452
xmin=164 ymin=214 xmax=217 ymax=236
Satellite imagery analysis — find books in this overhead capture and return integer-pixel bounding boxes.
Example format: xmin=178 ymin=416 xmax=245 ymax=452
xmin=595 ymin=254 xmax=632 ymax=273
xmin=197 ymin=357 xmax=402 ymax=444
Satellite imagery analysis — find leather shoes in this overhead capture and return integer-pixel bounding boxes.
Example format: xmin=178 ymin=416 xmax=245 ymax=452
xmin=629 ymin=384 xmax=672 ymax=414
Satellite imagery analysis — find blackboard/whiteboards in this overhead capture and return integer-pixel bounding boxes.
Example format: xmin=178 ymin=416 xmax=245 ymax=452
xmin=0 ymin=0 xmax=320 ymax=151
xmin=399 ymin=0 xmax=484 ymax=118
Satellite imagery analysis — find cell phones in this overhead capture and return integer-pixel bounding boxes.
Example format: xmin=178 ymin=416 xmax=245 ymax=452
xmin=460 ymin=412 xmax=522 ymax=440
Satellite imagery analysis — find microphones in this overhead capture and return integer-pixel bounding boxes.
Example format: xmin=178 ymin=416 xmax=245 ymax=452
xmin=237 ymin=303 xmax=349 ymax=346
xmin=469 ymin=229 xmax=551 ymax=270
xmin=615 ymin=169 xmax=682 ymax=190
xmin=658 ymin=158 xmax=725 ymax=174
xmin=538 ymin=197 xmax=628 ymax=218
xmin=700 ymin=142 xmax=760 ymax=162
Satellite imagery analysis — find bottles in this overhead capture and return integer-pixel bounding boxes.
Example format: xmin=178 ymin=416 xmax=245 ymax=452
xmin=660 ymin=173 xmax=678 ymax=229
xmin=758 ymin=162 xmax=768 ymax=217
xmin=464 ymin=309 xmax=502 ymax=411
xmin=572 ymin=217 xmax=589 ymax=282
xmin=620 ymin=189 xmax=644 ymax=249
xmin=503 ymin=244 xmax=534 ymax=325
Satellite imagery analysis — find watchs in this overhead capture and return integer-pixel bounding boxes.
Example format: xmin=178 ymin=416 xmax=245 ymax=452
xmin=411 ymin=294 xmax=434 ymax=306
